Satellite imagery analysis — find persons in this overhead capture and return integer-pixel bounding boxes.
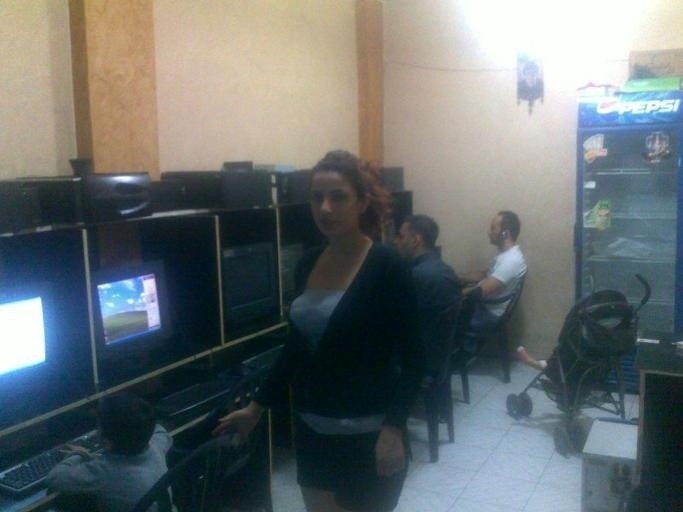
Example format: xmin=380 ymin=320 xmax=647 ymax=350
xmin=210 ymin=146 xmax=425 ymax=510
xmin=45 ymin=393 xmax=176 ymax=509
xmin=395 ymin=212 xmax=463 ymax=389
xmin=457 ymin=209 xmax=523 ymax=333
xmin=514 ymin=344 xmax=549 ymax=370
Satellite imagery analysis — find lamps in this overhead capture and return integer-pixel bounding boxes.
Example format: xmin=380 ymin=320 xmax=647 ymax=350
xmin=515 ymin=52 xmax=543 ymax=116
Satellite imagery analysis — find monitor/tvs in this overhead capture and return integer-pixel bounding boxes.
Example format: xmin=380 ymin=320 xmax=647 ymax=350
xmin=83 ymin=172 xmax=153 ymax=221
xmin=91 ymin=258 xmax=174 ymax=362
xmin=221 ymin=242 xmax=276 ymax=324
xmin=0 ymin=283 xmax=58 ymax=385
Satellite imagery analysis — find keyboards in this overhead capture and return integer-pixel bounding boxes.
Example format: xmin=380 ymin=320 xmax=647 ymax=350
xmin=1 ymin=431 xmax=107 ymax=496
xmin=241 ymin=344 xmax=285 ymax=369
xmin=152 ymin=379 xmax=230 ymax=417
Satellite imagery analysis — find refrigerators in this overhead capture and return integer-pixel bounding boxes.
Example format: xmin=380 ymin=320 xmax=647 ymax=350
xmin=576 ymin=80 xmax=683 ymax=396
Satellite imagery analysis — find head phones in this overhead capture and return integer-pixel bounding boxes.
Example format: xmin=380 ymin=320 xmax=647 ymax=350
xmin=498 ymin=212 xmax=512 ymax=241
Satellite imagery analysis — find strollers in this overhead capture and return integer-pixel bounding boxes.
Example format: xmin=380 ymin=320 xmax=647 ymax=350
xmin=507 ymin=272 xmax=652 ymax=458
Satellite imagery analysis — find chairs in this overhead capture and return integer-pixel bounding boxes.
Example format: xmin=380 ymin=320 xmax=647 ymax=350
xmin=411 ymin=298 xmax=465 ymax=463
xmin=164 ymin=365 xmax=270 ymax=471
xmin=469 ymin=269 xmax=528 ymax=382
xmin=134 ymin=433 xmax=233 ymax=512
xmin=454 ymin=287 xmax=483 ymax=405
